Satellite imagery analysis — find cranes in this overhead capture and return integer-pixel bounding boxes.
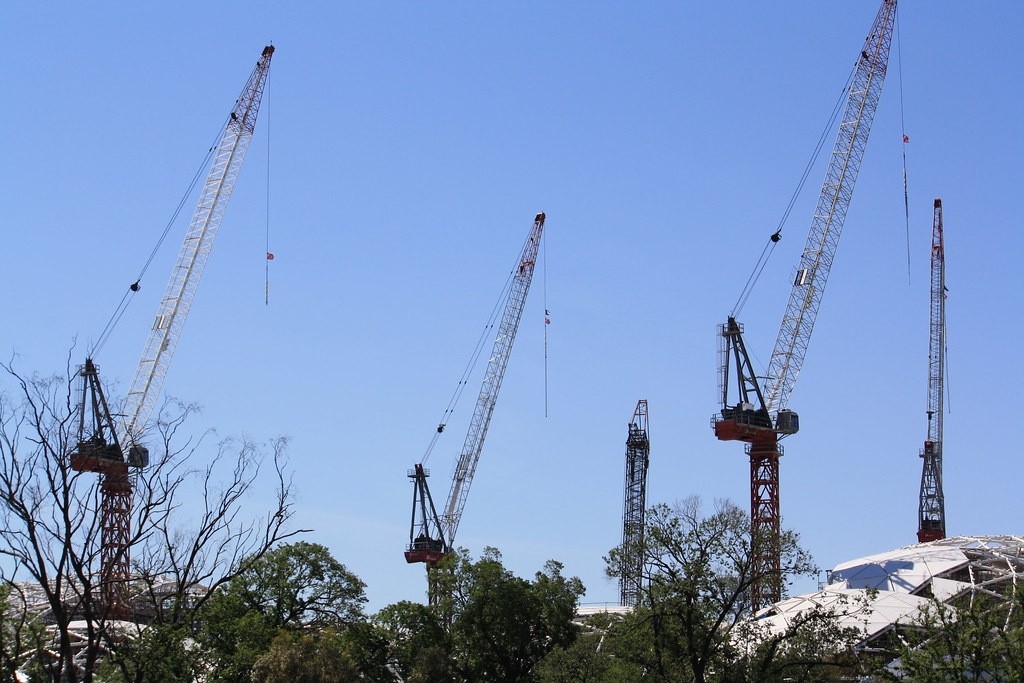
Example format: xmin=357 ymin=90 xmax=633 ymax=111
xmin=24 ymin=37 xmax=281 ymax=680
xmin=403 ymin=214 xmax=552 ymax=619
xmin=705 ymin=0 xmax=908 ymax=612
xmin=915 ymin=198 xmax=948 ymax=546
xmin=617 ymin=397 xmax=651 ymax=611
